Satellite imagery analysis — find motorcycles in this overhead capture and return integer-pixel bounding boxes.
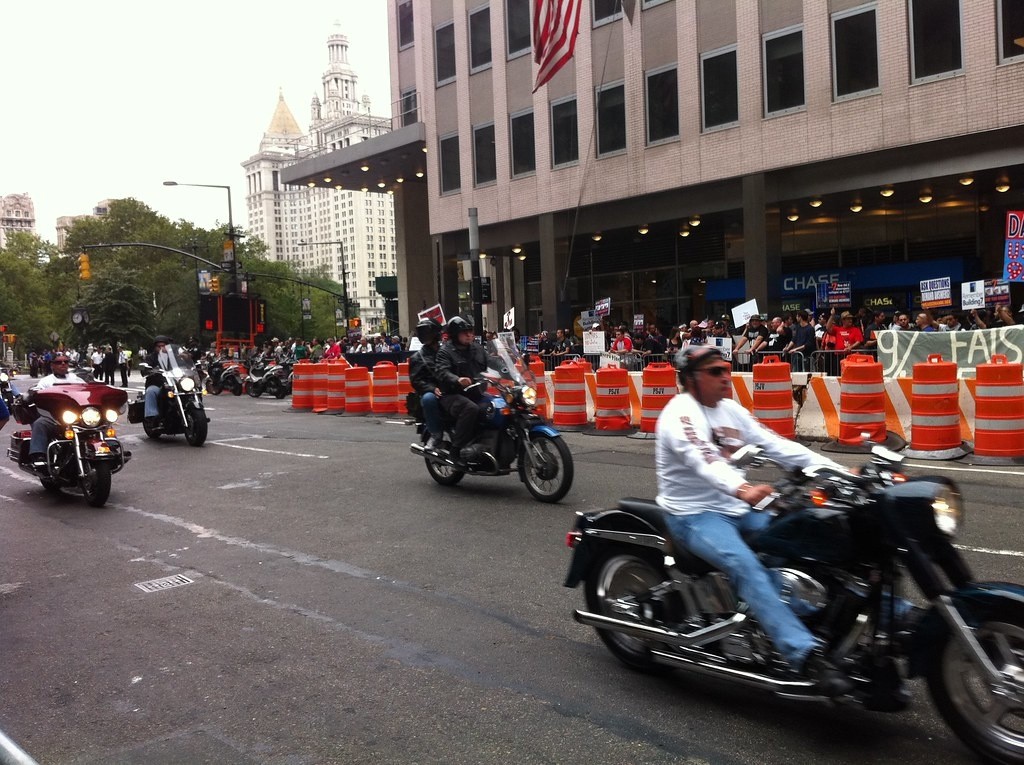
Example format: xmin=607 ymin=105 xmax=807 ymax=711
xmin=400 ymin=339 xmax=574 ymax=503
xmin=562 ymin=445 xmax=1024 ymax=765
xmin=127 ymin=343 xmax=210 ymax=446
xmin=0 ymin=366 xmax=132 ymax=507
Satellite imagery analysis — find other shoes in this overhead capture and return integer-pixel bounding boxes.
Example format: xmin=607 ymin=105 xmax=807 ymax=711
xmin=150 ymin=416 xmax=165 ymax=431
xmin=119 ymin=385 xmax=129 ymax=388
xmin=110 ymin=447 xmax=132 ymax=470
xmin=801 ymin=646 xmax=874 ymax=689
xmin=33 ymin=456 xmax=48 ymax=469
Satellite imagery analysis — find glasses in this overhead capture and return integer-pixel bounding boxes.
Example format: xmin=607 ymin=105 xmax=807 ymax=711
xmin=692 ymin=367 xmax=728 ymax=377
xmin=51 ymin=359 xmax=70 ymax=365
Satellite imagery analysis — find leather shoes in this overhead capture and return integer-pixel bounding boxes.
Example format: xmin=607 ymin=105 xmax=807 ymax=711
xmin=449 ymin=448 xmax=467 ymax=468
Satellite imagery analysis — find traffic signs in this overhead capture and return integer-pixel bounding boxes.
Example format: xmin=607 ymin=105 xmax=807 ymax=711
xmin=220 ymin=261 xmax=243 ymax=270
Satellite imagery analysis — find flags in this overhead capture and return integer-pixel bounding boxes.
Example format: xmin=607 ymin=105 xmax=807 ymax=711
xmin=533 ymin=0 xmax=582 ymax=94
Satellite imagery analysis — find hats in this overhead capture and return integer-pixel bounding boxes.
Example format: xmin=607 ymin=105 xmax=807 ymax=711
xmin=51 ymin=352 xmax=67 ymax=359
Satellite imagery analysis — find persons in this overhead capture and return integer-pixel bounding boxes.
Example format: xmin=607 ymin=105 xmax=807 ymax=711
xmin=410 ymin=318 xmax=443 ymax=449
xmin=139 ymin=336 xmax=203 ymax=433
xmin=655 ymin=344 xmax=916 ymax=695
xmin=485 ymin=305 xmax=1024 ymax=374
xmin=183 ymin=332 xmax=408 ymax=364
xmin=434 ymin=316 xmax=515 ymax=462
xmin=29 ymin=355 xmax=131 ymax=468
xmin=10 ymin=382 xmax=19 ymax=398
xmin=28 ymin=344 xmax=147 ymax=387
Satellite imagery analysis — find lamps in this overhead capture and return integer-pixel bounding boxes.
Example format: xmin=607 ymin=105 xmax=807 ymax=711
xmin=847 ymin=189 xmax=863 ymax=213
xmin=591 ymin=231 xmax=602 ymax=242
xmin=386 ymin=182 xmax=394 ymax=194
xmin=322 ymin=171 xmax=331 ymax=183
xmin=516 ymin=250 xmax=527 ymax=261
xmin=510 ymin=243 xmax=523 ymax=254
xmin=809 ymin=194 xmax=823 ymax=208
xmin=879 ymin=183 xmax=894 ymax=197
xmin=395 ymin=166 xmax=404 ymax=183
xmin=361 ymin=179 xmax=368 ymax=192
xmin=375 ymin=172 xmax=386 ymax=188
xmin=679 ymin=220 xmax=690 ymax=236
xmin=416 ymin=162 xmax=424 ymax=178
xmin=917 ymin=179 xmax=934 ymax=203
xmin=307 ymin=177 xmax=316 ymax=187
xmin=478 ymin=248 xmax=486 ymax=259
xmin=688 ymin=215 xmax=701 ymax=227
xmin=334 ymin=179 xmax=342 ymax=190
xmin=420 ymin=144 xmax=427 ymax=153
xmin=785 ymin=200 xmax=800 ymax=221
xmin=956 ymin=171 xmax=974 ymax=186
xmin=995 ymin=166 xmax=1011 ymax=193
xmin=637 ymin=223 xmax=649 ymax=234
xmin=978 ymin=200 xmax=992 ymax=212
xmin=360 ymin=159 xmax=370 ymax=171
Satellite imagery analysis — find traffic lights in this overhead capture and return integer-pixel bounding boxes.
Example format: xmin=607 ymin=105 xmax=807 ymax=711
xmin=208 ymin=278 xmax=220 ymax=293
xmin=354 ymin=320 xmax=361 ymax=327
xmin=3 ymin=334 xmax=17 ymax=343
xmin=78 ymin=255 xmax=90 ymax=281
xmin=0 ymin=326 xmax=8 ymax=333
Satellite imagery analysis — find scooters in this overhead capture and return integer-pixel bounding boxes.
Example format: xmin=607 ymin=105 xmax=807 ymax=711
xmin=197 ymin=350 xmax=298 ymax=399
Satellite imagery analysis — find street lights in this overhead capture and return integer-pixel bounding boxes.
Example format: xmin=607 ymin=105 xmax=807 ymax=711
xmin=297 ymin=239 xmax=349 ymax=337
xmin=163 ymin=182 xmax=242 ymax=360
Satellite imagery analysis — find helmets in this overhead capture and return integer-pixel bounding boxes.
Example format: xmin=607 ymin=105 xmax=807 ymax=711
xmin=445 ymin=315 xmax=474 ymax=336
xmin=415 ymin=316 xmax=443 ymax=331
xmin=675 ymin=345 xmax=733 ymax=369
xmin=153 ymin=335 xmax=170 ymax=344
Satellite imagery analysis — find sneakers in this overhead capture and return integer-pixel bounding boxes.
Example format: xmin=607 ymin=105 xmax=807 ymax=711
xmin=423 ymin=436 xmax=444 ymax=451
